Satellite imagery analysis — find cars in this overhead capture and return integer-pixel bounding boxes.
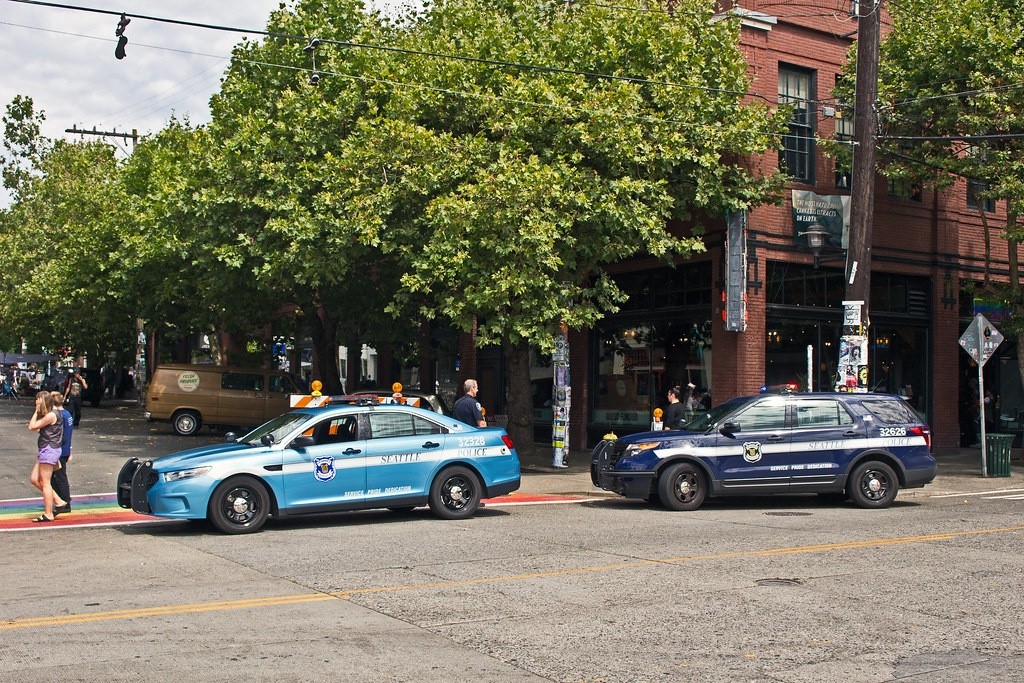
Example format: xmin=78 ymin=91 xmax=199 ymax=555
xmin=592 ymin=383 xmax=936 ymax=510
xmin=116 ymin=395 xmax=523 ymax=535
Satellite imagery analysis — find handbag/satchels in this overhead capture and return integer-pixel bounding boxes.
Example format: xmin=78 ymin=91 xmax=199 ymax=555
xmin=54 ymin=460 xmax=62 ymax=472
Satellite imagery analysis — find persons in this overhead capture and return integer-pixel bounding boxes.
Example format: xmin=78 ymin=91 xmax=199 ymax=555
xmin=452 ymin=379 xmax=487 ymax=428
xmin=759 ymin=408 xmax=781 ymax=429
xmin=0 ymin=359 xmax=135 ymax=429
xmin=27 ymin=391 xmax=69 ymax=522
xmin=960 ymin=375 xmax=997 ymax=450
xmin=662 ymin=383 xmax=711 ymax=431
xmin=359 ymin=376 xmax=377 ymax=389
xmin=50 ymin=391 xmax=75 ymax=513
xmin=838 ymin=385 xmax=848 ymax=393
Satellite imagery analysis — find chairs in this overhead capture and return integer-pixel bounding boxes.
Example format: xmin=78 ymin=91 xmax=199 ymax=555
xmin=336 ymin=424 xmax=352 ymax=442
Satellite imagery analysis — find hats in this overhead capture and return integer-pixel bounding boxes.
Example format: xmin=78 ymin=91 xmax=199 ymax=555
xmin=73 ymin=368 xmax=81 ymax=373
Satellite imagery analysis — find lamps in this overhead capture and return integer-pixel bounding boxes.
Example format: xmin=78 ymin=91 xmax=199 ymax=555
xmin=799 ymin=217 xmax=847 ymax=271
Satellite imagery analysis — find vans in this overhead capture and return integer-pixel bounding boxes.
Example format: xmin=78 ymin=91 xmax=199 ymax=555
xmin=143 ymin=363 xmax=312 ymax=435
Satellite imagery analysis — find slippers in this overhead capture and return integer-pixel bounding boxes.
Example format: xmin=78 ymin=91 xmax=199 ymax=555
xmin=53 ymin=505 xmax=66 ymax=517
xmin=33 ymin=514 xmax=54 ymax=522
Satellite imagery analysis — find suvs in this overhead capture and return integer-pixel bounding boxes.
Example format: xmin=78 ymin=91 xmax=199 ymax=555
xmin=41 ymin=365 xmax=103 ymax=405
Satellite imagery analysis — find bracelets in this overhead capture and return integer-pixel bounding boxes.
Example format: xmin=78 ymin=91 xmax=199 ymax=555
xmin=35 ymin=410 xmax=39 ymax=415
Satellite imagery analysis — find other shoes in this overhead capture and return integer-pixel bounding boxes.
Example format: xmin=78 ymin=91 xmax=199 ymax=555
xmin=74 ymin=426 xmax=78 ymax=430
xmin=60 ymin=508 xmax=71 ymax=513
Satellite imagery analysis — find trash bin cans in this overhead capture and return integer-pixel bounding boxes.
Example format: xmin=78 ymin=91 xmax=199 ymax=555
xmin=978 ymin=432 xmax=1017 ymax=477
